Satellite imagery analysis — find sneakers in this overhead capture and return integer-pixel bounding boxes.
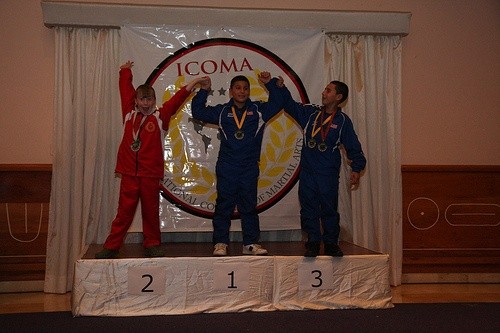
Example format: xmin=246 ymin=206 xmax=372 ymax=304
xmin=148 ymin=247 xmax=164 ymax=257
xmin=324 ymin=244 xmax=343 ymax=257
xmin=304 ymin=246 xmax=320 ymax=257
xmin=214 ymin=243 xmax=227 ymax=255
xmin=242 ymin=243 xmax=268 ymax=254
xmin=96 ymin=248 xmax=117 ymax=257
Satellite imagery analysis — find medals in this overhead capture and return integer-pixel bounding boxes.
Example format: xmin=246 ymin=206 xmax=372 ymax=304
xmin=318 ymin=143 xmax=327 ymax=152
xmin=235 ymin=131 xmax=244 ymax=140
xmin=308 ymin=140 xmax=317 ymax=148
xmin=130 ymin=142 xmax=140 ymax=152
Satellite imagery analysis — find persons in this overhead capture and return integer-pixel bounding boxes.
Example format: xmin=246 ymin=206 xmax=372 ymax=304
xmin=94 ymin=60 xmax=207 ymax=260
xmin=191 ymin=74 xmax=288 ymax=255
xmin=258 ymin=71 xmax=367 ymax=257
